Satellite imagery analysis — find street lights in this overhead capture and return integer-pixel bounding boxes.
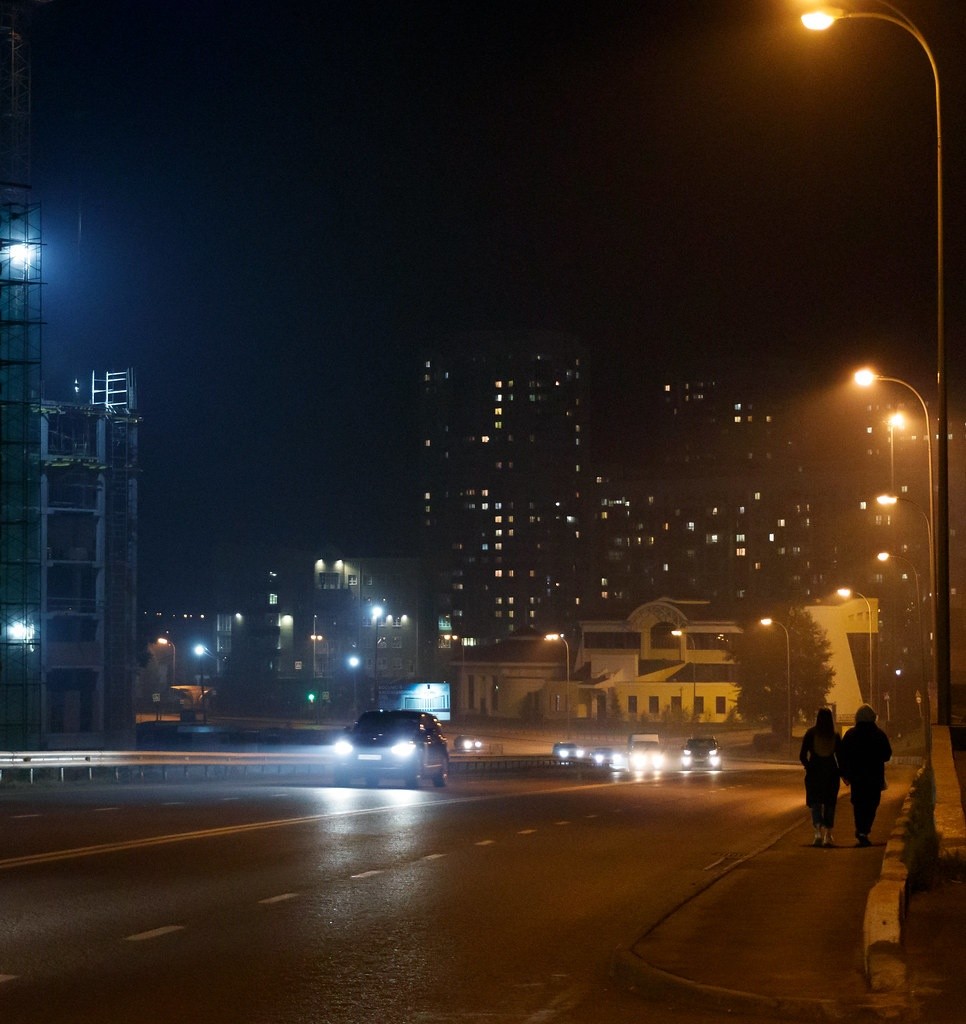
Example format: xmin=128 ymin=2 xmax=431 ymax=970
xmin=760 ymin=617 xmax=792 ymax=762
xmin=156 ymin=637 xmax=177 ymax=695
xmin=671 ymin=629 xmax=696 ymax=712
xmin=544 ymin=631 xmax=571 ymax=732
xmin=836 ymin=587 xmax=873 ymax=708
xmin=195 ymin=643 xmax=207 ymax=722
xmin=854 ymin=367 xmax=934 ymax=755
xmin=372 ymin=606 xmax=382 ymax=700
xmin=799 ymin=1 xmax=952 ymax=725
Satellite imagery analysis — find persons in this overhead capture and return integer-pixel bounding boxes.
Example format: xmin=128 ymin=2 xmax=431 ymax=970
xmin=840 ymin=704 xmax=892 ymax=846
xmin=799 ymin=707 xmax=841 ymax=847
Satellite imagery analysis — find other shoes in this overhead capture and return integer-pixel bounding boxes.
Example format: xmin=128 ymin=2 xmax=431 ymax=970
xmin=825 ymin=836 xmax=834 ymax=846
xmin=814 ymin=833 xmax=822 ymax=846
xmin=856 ymin=831 xmax=869 ymax=844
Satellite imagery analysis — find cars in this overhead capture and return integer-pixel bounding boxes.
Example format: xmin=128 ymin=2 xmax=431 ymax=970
xmin=336 ymin=710 xmax=451 ymax=788
xmin=454 ymin=735 xmax=482 ymax=750
xmin=554 ymin=737 xmax=722 ymax=770
